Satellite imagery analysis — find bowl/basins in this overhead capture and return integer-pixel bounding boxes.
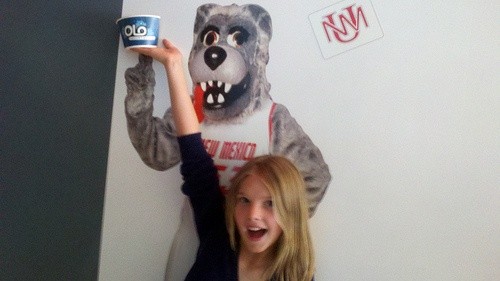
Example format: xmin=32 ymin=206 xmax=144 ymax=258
xmin=116 ymin=15 xmax=160 ymax=50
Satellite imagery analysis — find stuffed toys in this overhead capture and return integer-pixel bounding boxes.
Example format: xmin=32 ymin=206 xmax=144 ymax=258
xmin=123 ymin=3 xmax=332 ymax=281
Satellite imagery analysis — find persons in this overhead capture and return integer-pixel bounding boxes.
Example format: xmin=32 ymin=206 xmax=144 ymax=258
xmin=130 ymin=39 xmax=316 ymax=281
